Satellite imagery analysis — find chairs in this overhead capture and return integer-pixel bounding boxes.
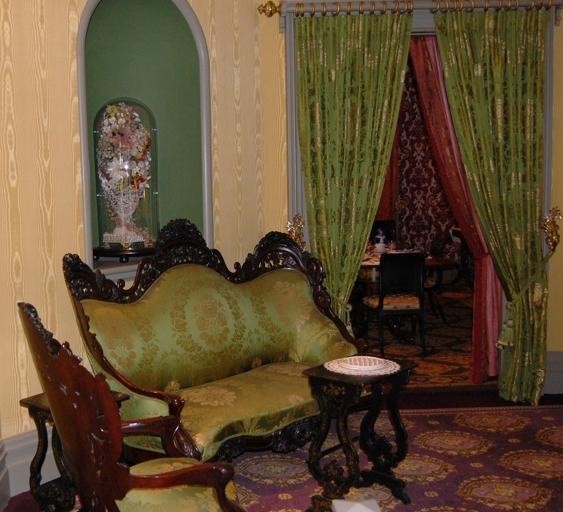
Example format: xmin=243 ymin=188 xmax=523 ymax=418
xmin=17 ymin=301 xmax=245 ymax=512
xmin=351 ymin=214 xmax=474 ymax=354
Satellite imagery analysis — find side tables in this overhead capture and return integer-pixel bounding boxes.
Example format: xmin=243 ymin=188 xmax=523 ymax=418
xmin=19 ymin=390 xmax=129 ymax=512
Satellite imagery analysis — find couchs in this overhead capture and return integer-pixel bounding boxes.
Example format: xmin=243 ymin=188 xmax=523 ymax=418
xmin=62 ymin=217 xmax=369 ymax=464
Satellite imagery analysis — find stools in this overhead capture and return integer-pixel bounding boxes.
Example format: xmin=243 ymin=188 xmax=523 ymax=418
xmin=300 ymin=352 xmax=417 ymax=512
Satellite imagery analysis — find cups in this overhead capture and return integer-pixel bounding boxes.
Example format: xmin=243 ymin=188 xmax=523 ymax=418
xmin=388 ymin=241 xmax=396 ymax=250
